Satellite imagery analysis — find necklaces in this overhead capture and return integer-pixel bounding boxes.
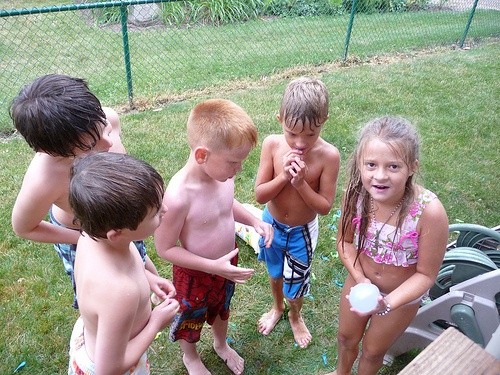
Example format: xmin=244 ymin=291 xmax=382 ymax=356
xmin=370 ymin=194 xmax=405 ymax=256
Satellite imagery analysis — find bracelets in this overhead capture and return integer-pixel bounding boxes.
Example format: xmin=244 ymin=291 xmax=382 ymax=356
xmin=375 ymin=297 xmax=391 ymax=316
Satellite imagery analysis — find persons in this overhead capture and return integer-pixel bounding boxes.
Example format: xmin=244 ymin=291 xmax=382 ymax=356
xmin=324 ymin=116 xmax=449 ymax=375
xmin=8 ymin=74 xmax=160 ymax=309
xmin=67 ymin=152 xmax=181 ymax=375
xmin=254 ymin=78 xmax=340 ymax=349
xmin=154 ymin=99 xmax=275 ymax=375
